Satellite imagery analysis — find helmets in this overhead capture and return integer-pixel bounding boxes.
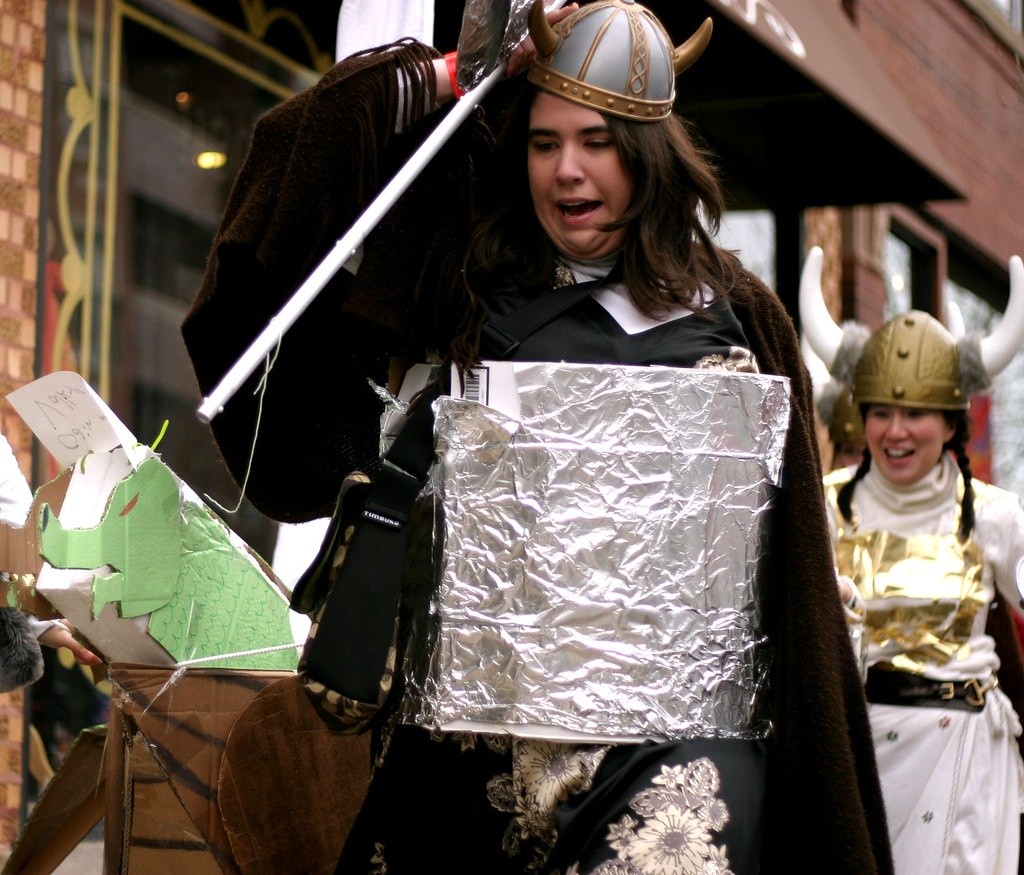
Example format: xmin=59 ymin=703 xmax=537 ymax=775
xmin=524 ymin=0 xmax=714 ymax=124
xmin=798 ymin=244 xmax=1023 ymax=411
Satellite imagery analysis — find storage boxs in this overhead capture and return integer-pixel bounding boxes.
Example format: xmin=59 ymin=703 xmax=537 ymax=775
xmin=33 ymin=443 xmax=308 ymax=675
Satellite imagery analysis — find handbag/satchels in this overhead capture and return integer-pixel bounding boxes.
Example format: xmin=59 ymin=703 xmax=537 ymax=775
xmin=288 ymin=368 xmax=453 ymax=734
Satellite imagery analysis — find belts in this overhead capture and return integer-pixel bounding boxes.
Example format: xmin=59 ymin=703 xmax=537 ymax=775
xmin=864 ymin=665 xmax=1001 ymax=712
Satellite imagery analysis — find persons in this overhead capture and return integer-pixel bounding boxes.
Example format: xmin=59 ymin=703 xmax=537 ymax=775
xmin=0 ymin=607 xmax=104 ymax=695
xmin=822 ymin=311 xmax=1024 ymax=875
xmin=177 ymin=4 xmax=893 ymax=875
